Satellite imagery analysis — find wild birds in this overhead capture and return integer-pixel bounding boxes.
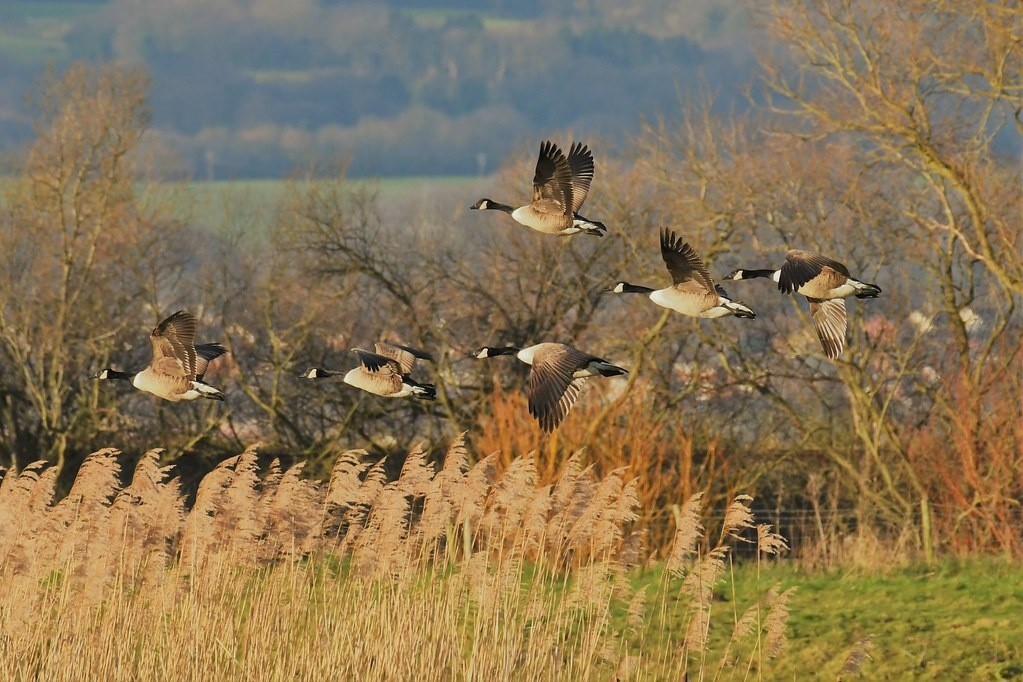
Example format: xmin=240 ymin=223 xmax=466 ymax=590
xmin=299 ymin=342 xmax=437 ymax=402
xmin=722 ymin=249 xmax=881 ymax=359
xmin=89 ymin=310 xmax=230 ymax=402
xmin=466 ymin=342 xmax=630 ymax=435
xmin=602 ymin=226 xmax=757 ymax=319
xmin=470 ymin=141 xmax=608 ymax=239
